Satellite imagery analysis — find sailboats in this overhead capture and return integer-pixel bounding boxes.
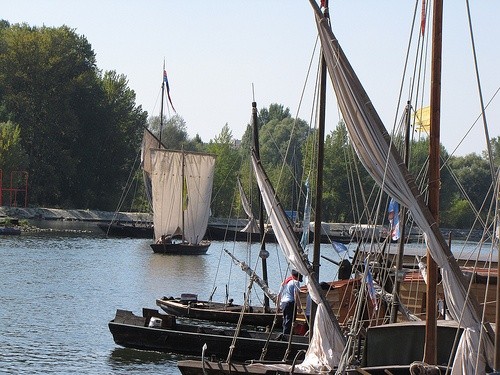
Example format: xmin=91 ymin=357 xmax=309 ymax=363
xmin=96 ymin=57 xmax=219 ymax=255
xmin=108 ymin=0 xmax=500 ymax=375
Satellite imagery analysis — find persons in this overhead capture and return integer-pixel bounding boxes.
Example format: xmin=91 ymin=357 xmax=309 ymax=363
xmin=277 ymin=270 xmax=312 ymax=336
